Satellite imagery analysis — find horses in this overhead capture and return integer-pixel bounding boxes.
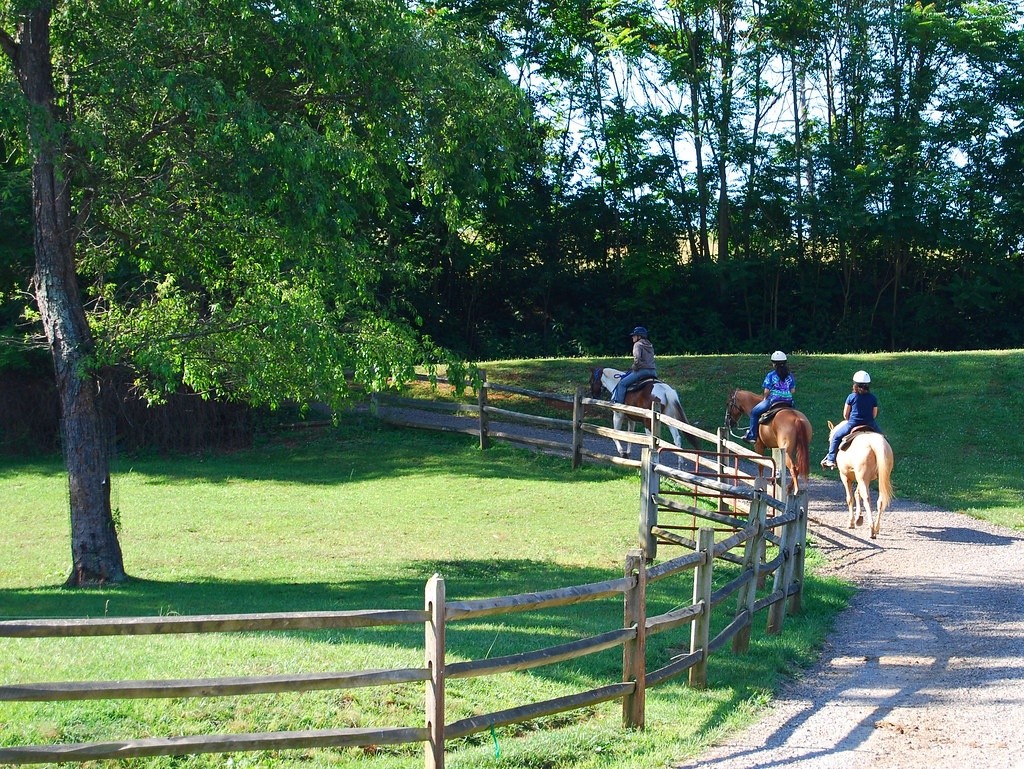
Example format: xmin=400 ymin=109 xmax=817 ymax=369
xmin=827 ymin=420 xmax=901 ymax=540
xmin=588 ymin=366 xmax=703 ymax=472
xmin=723 ymin=385 xmax=813 ymax=497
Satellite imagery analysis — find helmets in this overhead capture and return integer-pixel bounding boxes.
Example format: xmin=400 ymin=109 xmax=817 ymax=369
xmin=629 ymin=327 xmax=648 ymax=336
xmin=770 ymin=350 xmax=787 ymax=361
xmin=853 ymin=370 xmax=871 ymax=383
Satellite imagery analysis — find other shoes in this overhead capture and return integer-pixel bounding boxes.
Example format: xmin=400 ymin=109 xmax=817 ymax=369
xmin=741 ymin=435 xmax=756 ymax=443
xmin=823 ymin=460 xmax=836 ymax=467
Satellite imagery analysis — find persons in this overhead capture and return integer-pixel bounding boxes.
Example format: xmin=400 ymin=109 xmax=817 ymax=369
xmin=741 ymin=351 xmax=795 ymax=443
xmin=612 ymin=327 xmax=657 ymax=403
xmin=827 ymin=370 xmax=881 ymax=467
